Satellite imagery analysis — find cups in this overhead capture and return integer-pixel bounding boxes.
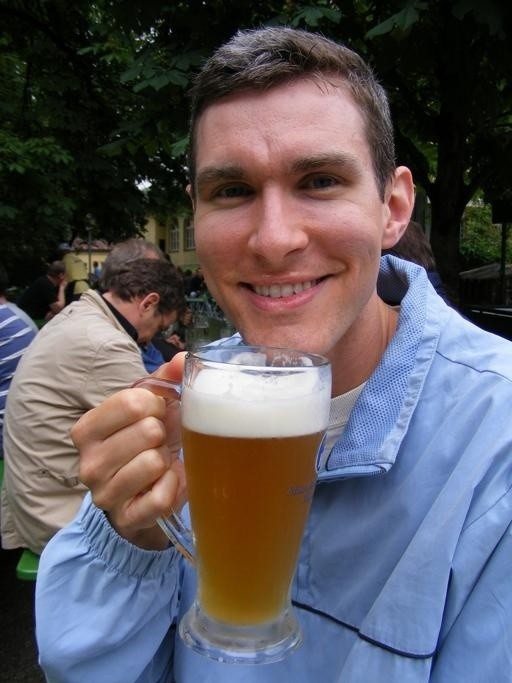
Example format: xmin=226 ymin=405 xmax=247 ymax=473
xmin=125 ymin=344 xmax=332 ymax=662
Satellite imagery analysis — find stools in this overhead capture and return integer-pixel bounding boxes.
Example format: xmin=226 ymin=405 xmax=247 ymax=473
xmin=14 ymin=548 xmax=40 ymax=611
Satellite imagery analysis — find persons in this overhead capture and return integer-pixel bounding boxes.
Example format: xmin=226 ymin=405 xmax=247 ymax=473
xmin=0 ymin=256 xmax=189 ymax=562
xmin=31 ymin=20 xmax=512 ymax=682
xmin=0 ymin=286 xmax=41 ymax=592
xmin=100 ymin=236 xmax=172 ymax=279
xmin=42 ymin=250 xmax=207 ymax=376
xmin=378 ymin=218 xmax=477 ymax=325
